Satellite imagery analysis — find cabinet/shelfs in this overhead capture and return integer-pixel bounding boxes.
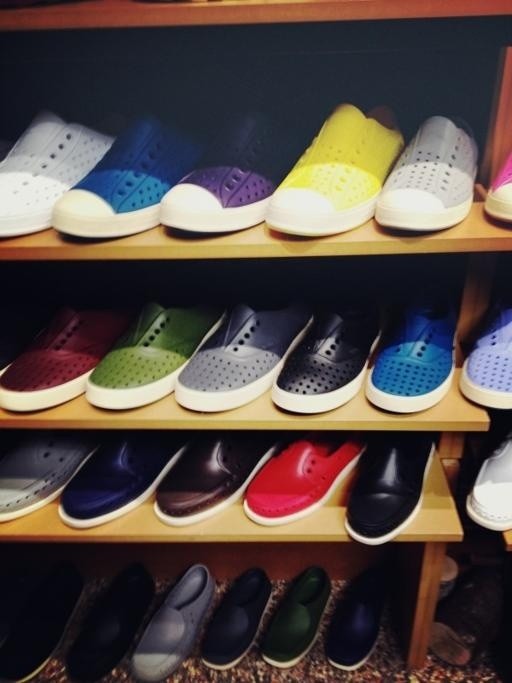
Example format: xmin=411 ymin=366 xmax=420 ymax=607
xmin=0 ymin=0 xmax=512 ymax=683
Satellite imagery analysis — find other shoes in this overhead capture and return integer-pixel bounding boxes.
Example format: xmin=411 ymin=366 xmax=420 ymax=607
xmin=0 ymin=552 xmax=385 ymax=682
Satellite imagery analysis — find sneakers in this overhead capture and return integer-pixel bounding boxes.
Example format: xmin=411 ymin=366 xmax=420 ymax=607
xmin=0 ymin=103 xmax=512 ymax=546
